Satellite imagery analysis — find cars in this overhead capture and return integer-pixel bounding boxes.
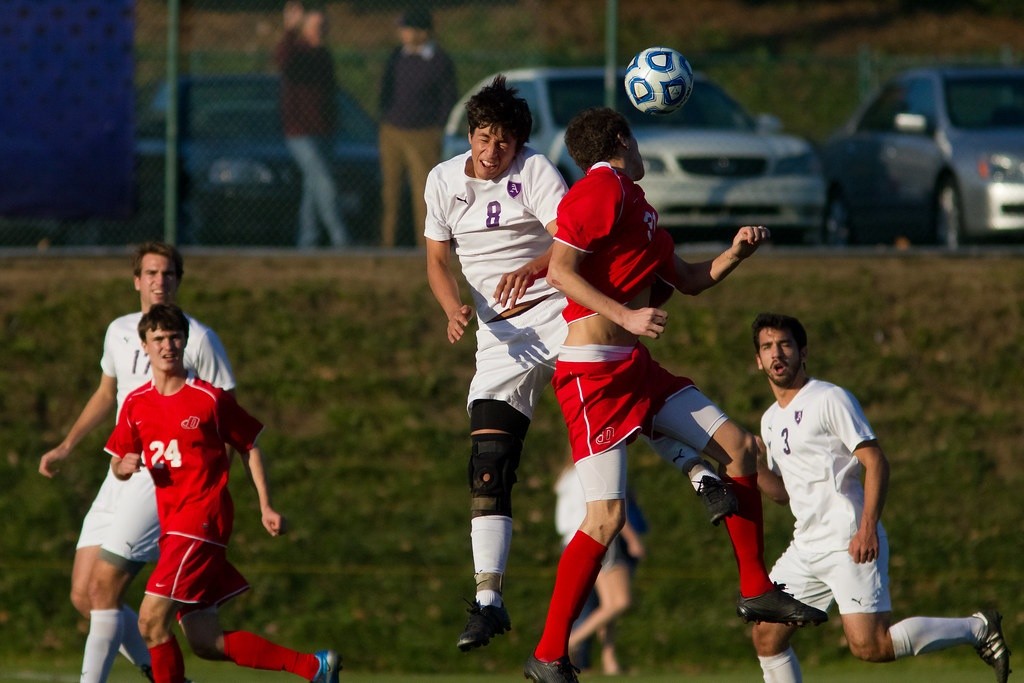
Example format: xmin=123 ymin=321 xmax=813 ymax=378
xmin=441 ymin=60 xmax=829 ymax=249
xmin=109 ymin=61 xmax=415 ymax=249
xmin=816 ymin=65 xmax=1024 ymax=252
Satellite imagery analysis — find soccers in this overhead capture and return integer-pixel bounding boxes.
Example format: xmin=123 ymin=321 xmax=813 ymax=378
xmin=624 ymin=46 xmax=694 ymax=118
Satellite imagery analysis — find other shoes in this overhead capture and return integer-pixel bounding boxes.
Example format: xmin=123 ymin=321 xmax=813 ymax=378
xmin=310 ymin=650 xmax=342 ymax=683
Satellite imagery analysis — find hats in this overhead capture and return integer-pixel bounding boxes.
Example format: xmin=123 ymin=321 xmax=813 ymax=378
xmin=401 ymin=7 xmax=433 ymax=30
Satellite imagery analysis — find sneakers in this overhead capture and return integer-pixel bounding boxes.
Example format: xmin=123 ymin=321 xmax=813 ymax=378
xmin=738 ymin=584 xmax=828 ymax=626
xmin=523 ymin=647 xmax=581 ymax=683
xmin=456 ymin=597 xmax=512 ymax=651
xmin=690 ymin=475 xmax=733 ymax=525
xmin=974 ymin=608 xmax=1012 ymax=683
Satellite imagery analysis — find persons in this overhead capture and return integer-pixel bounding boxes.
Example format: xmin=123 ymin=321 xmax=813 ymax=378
xmin=747 ymin=310 xmax=1013 ymax=683
xmin=423 ymin=77 xmax=803 ymax=683
xmin=271 ymin=0 xmax=352 ymax=253
xmin=38 ymin=243 xmax=338 ymax=682
xmin=381 ymin=1 xmax=456 ymax=247
